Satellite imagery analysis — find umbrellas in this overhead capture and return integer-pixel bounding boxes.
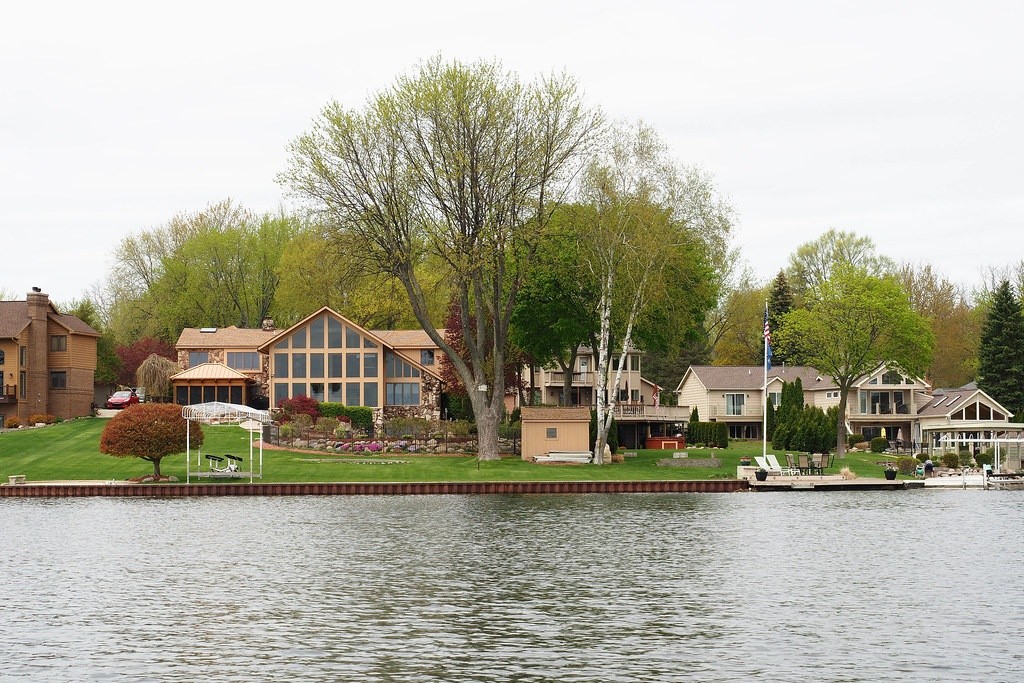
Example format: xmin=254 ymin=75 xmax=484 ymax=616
xmin=897 ymin=428 xmax=904 ymax=446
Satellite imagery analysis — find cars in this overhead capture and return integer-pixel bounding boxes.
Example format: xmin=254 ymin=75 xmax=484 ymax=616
xmin=136 ymin=386 xmax=147 ymax=403
xmin=107 ymin=391 xmax=137 ymax=409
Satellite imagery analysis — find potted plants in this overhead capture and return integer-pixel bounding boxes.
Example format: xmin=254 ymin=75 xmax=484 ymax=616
xmin=740 ymin=455 xmax=751 ymax=466
xmin=754 ymin=467 xmax=768 ymax=481
xmin=884 ymin=466 xmax=897 ymax=480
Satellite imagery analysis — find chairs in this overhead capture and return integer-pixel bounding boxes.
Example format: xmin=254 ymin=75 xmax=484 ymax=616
xmin=753 ymin=452 xmax=835 ymax=477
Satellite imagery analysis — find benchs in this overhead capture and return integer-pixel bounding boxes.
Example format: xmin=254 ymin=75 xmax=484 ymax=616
xmin=8 ymin=475 xmax=26 ymax=485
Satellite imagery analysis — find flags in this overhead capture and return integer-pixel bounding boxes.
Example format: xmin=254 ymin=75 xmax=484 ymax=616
xmin=764 ymin=310 xmax=770 ymax=345
xmin=652 ymin=392 xmax=659 ymax=410
xmin=767 ymin=343 xmax=773 ymax=370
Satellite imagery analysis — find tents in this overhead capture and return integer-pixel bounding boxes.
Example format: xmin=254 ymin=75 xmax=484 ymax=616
xmin=181 ymin=402 xmax=271 ymax=484
